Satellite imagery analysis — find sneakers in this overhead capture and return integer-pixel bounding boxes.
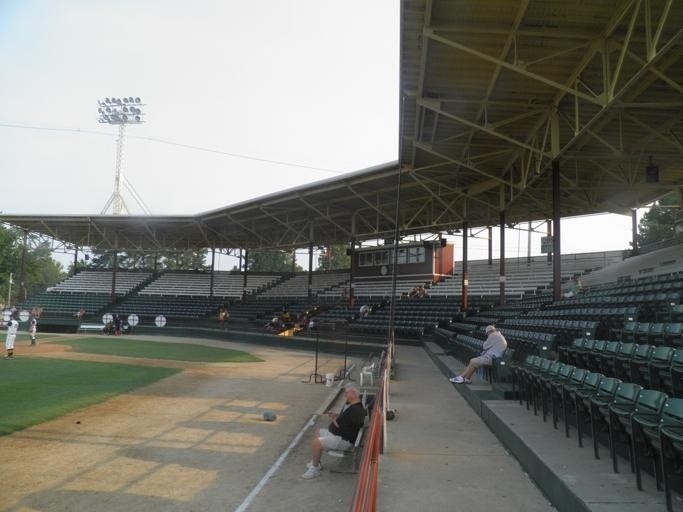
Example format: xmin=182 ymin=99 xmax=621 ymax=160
xmin=464 ymin=377 xmax=472 ymax=384
xmin=306 ymin=459 xmax=322 ymax=470
xmin=448 ymin=376 xmax=465 ymax=384
xmin=302 ymin=466 xmax=321 ymax=479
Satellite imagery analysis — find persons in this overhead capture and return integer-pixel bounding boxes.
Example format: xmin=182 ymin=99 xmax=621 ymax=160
xmin=0 ymin=304 xmax=44 ymax=360
xmin=302 ymin=381 xmax=365 ymax=479
xmin=449 ymin=325 xmax=508 ymax=383
xmin=217 ymin=281 xmax=434 ymax=336
xmin=101 ymin=312 xmax=124 ymax=336
xmin=561 ymin=278 xmax=582 ymax=298
xmin=73 ymin=308 xmax=87 ymax=319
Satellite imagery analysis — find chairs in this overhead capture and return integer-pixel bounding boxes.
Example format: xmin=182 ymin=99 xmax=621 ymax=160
xmin=14 ymin=271 xmax=682 ymax=510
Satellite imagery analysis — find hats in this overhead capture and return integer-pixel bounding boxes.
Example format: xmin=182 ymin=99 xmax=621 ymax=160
xmin=484 ymin=326 xmax=495 ymax=336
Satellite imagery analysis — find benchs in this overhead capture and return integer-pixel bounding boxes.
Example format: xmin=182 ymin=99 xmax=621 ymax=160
xmin=47 ymin=253 xmax=630 ymax=300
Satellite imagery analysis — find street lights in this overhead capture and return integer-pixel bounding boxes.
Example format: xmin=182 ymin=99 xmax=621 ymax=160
xmin=94 ymin=92 xmax=149 ymax=213
